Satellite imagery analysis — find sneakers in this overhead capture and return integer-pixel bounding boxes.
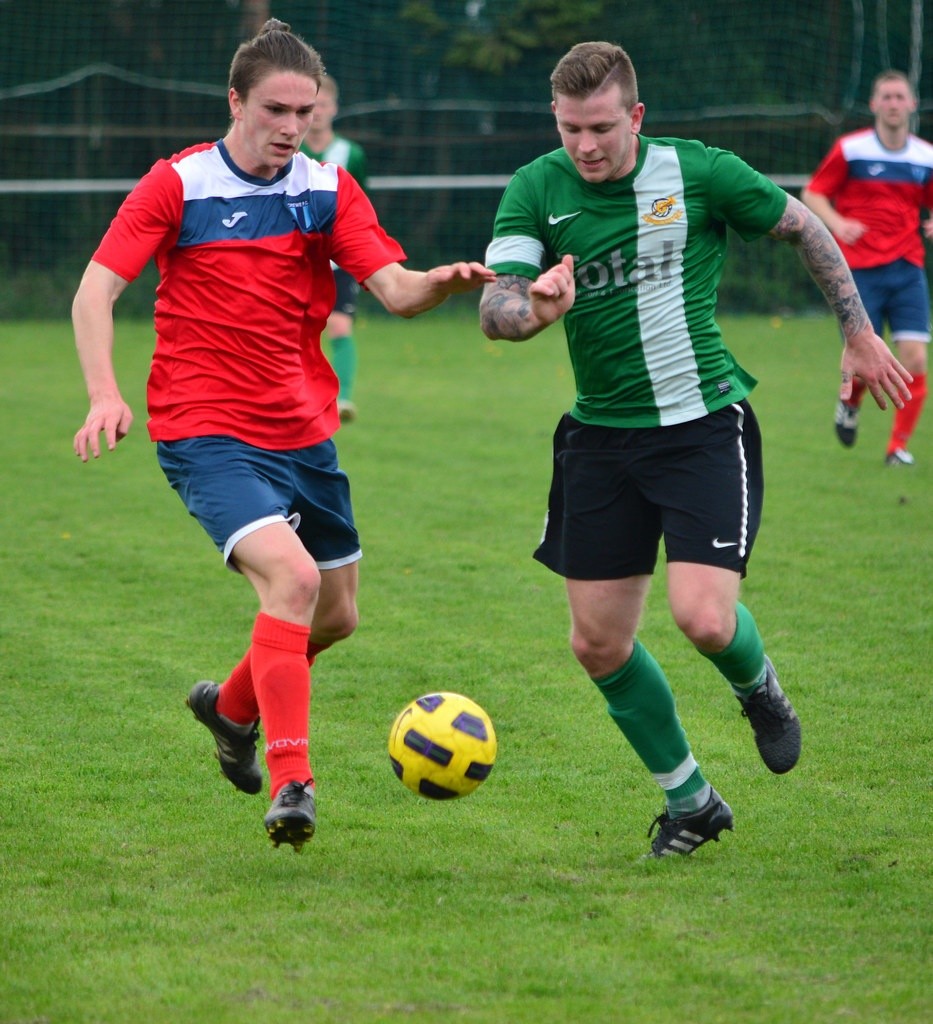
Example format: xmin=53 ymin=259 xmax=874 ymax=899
xmin=835 ymin=397 xmax=856 ymax=446
xmin=734 ymin=651 xmax=802 ymax=773
xmin=884 ymin=449 xmax=914 ymax=465
xmin=264 ymin=779 xmax=317 ymax=853
xmin=185 ymin=681 xmax=265 ymax=793
xmin=639 ymin=784 xmax=734 ymax=862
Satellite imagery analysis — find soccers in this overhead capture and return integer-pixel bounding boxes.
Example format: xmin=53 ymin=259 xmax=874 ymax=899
xmin=387 ymin=692 xmax=498 ymax=802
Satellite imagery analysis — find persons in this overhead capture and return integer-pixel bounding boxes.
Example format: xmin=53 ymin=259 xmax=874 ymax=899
xmin=73 ymin=18 xmax=499 ymax=849
xmin=799 ymin=67 xmax=933 ymax=466
xmin=296 ymin=72 xmax=373 ymax=423
xmin=479 ymin=42 xmax=916 ymax=859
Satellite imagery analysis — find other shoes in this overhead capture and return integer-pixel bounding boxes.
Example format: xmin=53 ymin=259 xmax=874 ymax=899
xmin=336 ymin=398 xmax=354 ymax=421
